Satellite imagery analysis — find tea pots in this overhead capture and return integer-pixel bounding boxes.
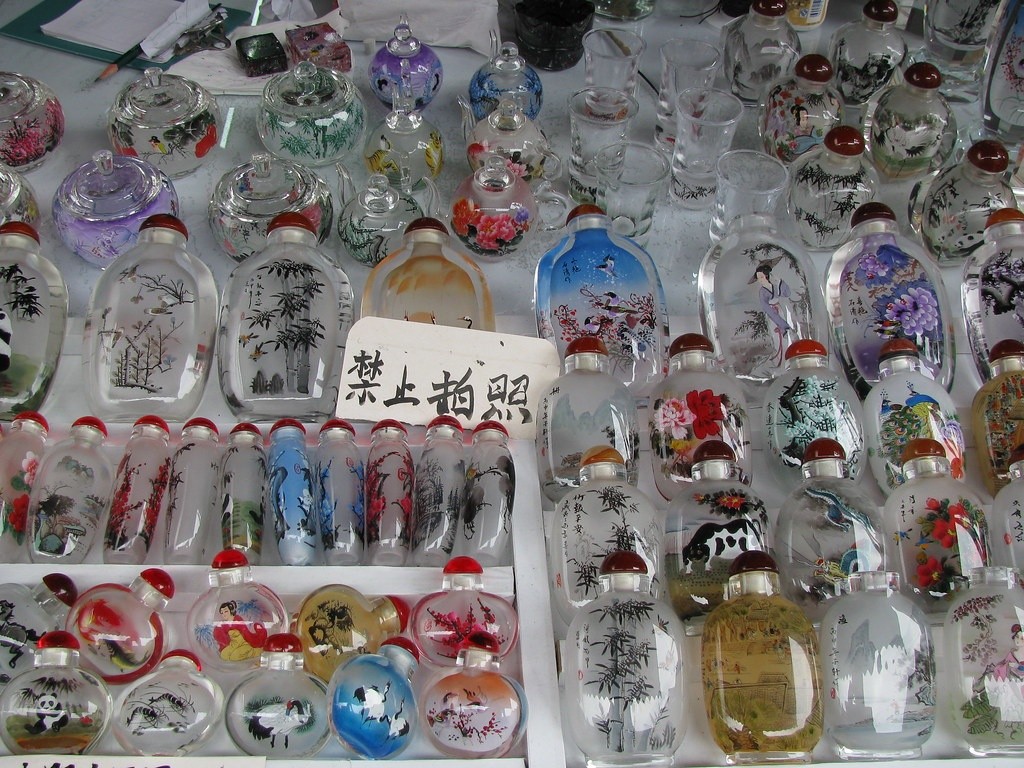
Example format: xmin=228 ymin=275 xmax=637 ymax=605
xmin=335 ymin=11 xmax=445 ymax=268
xmin=448 ymin=29 xmax=570 ymax=262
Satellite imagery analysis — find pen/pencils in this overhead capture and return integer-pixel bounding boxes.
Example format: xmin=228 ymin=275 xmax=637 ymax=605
xmin=95 ymin=38 xmax=144 ymax=83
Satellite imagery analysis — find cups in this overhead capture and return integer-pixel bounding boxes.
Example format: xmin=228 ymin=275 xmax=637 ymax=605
xmin=655 ymin=36 xmax=790 ymax=244
xmin=514 ymin=1 xmax=670 ymax=250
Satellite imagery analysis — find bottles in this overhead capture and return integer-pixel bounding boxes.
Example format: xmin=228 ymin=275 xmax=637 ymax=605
xmin=761 ymin=339 xmax=1024 ymax=500
xmin=0 ymin=570 xmax=225 ymax=757
xmin=187 ymin=550 xmax=421 ymax=760
xmin=663 ymin=441 xmax=825 ymax=767
xmin=829 ymin=0 xmax=1024 ymax=267
xmin=407 ymin=557 xmax=529 ymax=760
xmin=535 ymin=334 xmax=753 ymax=503
xmin=723 ymin=0 xmax=880 ymax=252
xmin=775 ymin=437 xmax=937 ymax=761
xmin=82 ymin=214 xmax=218 ymax=424
xmin=960 ymin=208 xmax=1024 ymax=385
xmin=360 ymin=218 xmax=496 ymax=333
xmin=883 ymin=438 xmax=1024 ymax=758
xmin=217 ymin=212 xmax=354 ymax=424
xmin=0 ymin=412 xmax=516 ymax=566
xmin=0 ymin=221 xmax=68 ymax=424
xmin=550 ymin=445 xmax=690 ymax=768
xmin=824 ymin=202 xmax=957 ymax=404
xmin=533 ymin=204 xmax=670 ymax=408
xmin=697 ymin=211 xmax=831 ymax=404
